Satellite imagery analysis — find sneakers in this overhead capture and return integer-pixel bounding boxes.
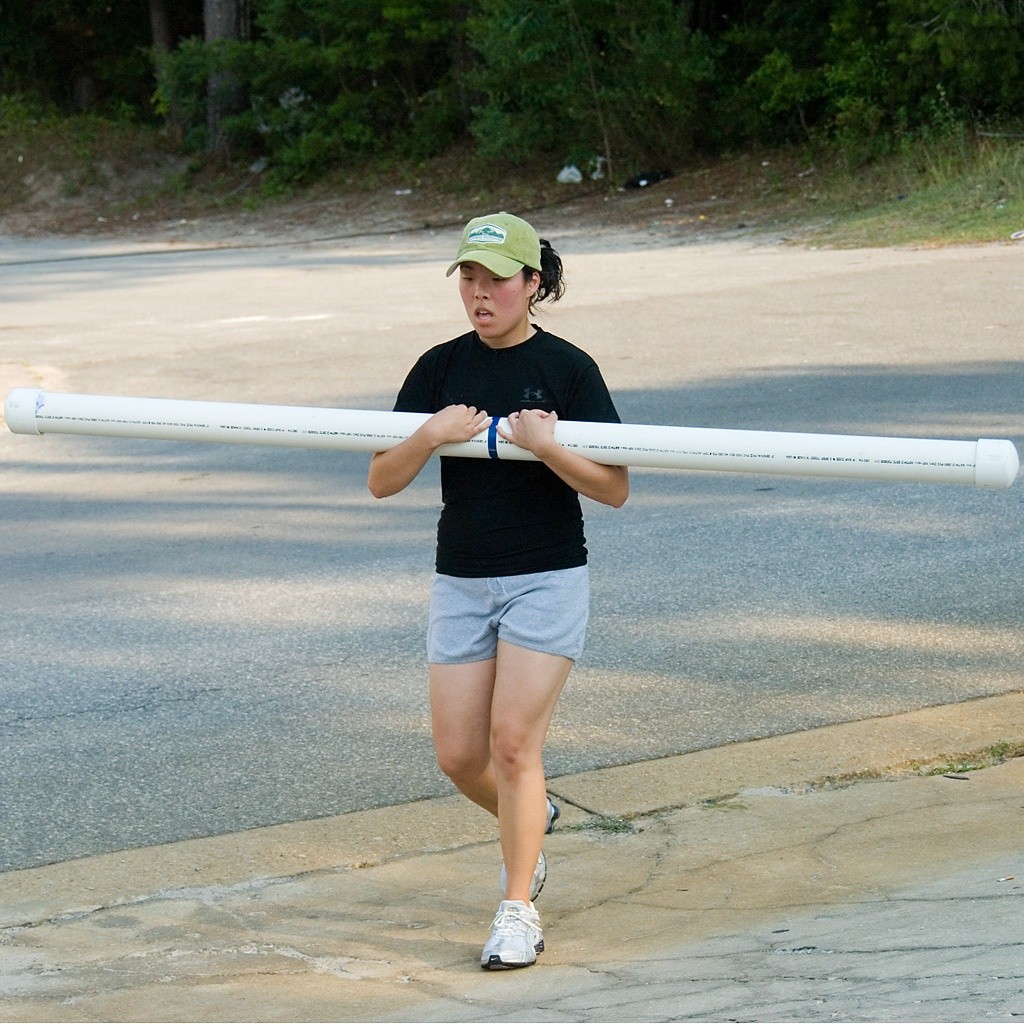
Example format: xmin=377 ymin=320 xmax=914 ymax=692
xmin=500 ymin=798 xmax=560 ymax=902
xmin=481 ymin=900 xmax=544 ymax=971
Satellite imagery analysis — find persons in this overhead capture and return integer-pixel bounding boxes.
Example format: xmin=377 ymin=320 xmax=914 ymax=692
xmin=367 ymin=212 xmax=630 ymax=971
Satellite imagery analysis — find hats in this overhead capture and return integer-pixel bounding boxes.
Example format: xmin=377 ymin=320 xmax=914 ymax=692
xmin=447 ymin=212 xmax=542 ymax=278
